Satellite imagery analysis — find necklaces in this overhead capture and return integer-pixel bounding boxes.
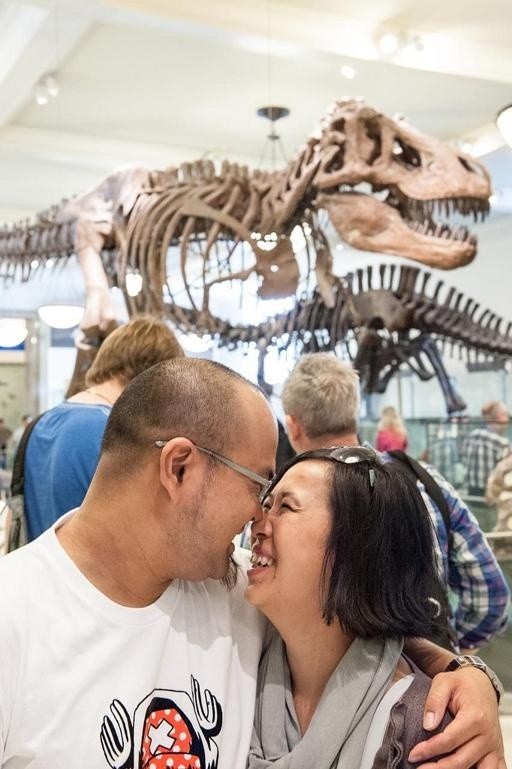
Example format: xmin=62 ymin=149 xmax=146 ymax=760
xmin=83 ymin=388 xmax=113 ymax=404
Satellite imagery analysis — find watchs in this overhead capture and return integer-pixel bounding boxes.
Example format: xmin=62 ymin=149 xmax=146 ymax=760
xmin=445 ymin=655 xmax=503 ymax=706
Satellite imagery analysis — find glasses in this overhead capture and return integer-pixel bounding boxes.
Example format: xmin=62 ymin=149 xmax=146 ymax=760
xmin=325 ymin=444 xmax=381 ymax=506
xmin=153 ymin=439 xmax=278 ymax=498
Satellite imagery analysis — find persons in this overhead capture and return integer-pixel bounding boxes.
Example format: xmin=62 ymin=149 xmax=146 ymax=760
xmin=428 ymin=401 xmax=470 ymax=484
xmin=22 ymin=316 xmax=185 ymax=542
xmin=461 ymin=402 xmax=512 ymax=496
xmin=280 ymin=352 xmax=511 ymax=656
xmin=0 ymin=357 xmax=507 ymax=769
xmin=9 ymin=415 xmax=32 ymax=450
xmin=376 ymin=406 xmax=408 ymax=452
xmin=0 ymin=419 xmax=13 ymax=469
xmin=244 ymin=446 xmax=477 ymax=769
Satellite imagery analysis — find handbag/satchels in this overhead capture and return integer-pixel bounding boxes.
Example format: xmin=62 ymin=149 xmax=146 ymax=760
xmin=0 ymin=408 xmax=50 ymax=556
